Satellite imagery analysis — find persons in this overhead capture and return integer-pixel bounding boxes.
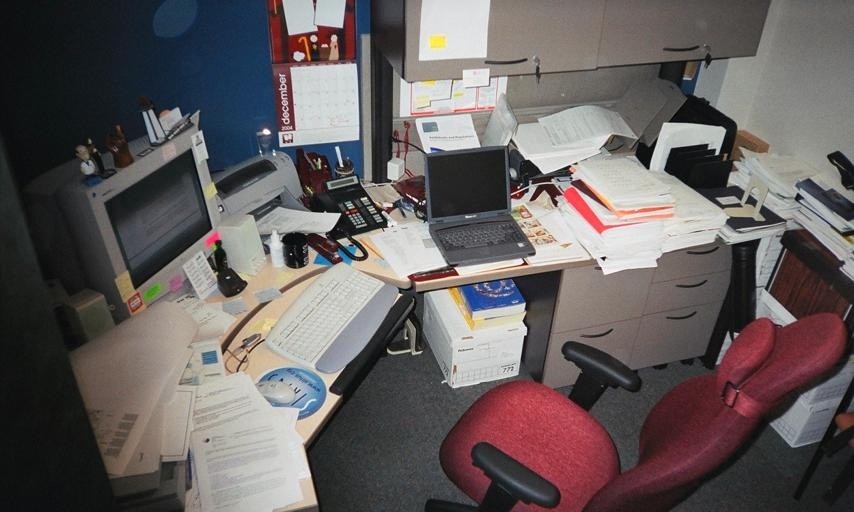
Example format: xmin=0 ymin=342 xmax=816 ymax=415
xmin=72 ymin=120 xmax=134 ymax=188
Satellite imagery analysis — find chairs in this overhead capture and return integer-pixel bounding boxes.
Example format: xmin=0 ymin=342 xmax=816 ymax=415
xmin=425 ymin=313 xmax=849 ymax=510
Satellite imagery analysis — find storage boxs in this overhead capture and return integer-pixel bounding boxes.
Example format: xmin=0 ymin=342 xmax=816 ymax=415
xmin=714 ymin=223 xmax=854 ymax=448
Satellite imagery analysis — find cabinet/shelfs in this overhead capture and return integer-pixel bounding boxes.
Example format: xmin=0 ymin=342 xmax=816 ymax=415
xmin=514 ymin=240 xmax=738 ymax=389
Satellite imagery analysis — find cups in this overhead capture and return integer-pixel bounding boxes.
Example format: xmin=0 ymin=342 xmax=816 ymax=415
xmin=105 ymin=138 xmax=134 ymax=168
xmin=334 ymin=160 xmax=355 ymax=180
xmin=281 ymin=233 xmax=309 ymax=268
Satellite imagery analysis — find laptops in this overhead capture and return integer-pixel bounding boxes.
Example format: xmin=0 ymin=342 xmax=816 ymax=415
xmin=424 ymin=146 xmax=536 ymax=266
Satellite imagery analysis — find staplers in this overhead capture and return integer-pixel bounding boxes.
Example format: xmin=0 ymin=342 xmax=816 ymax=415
xmin=306 ymin=233 xmax=343 ymax=264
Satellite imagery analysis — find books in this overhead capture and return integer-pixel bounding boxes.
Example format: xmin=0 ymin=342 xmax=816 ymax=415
xmin=456 ymin=277 xmax=527 ymax=315
xmin=482 ymin=93 xmax=518 ymax=150
xmin=449 ymin=287 xmax=527 ymax=332
xmin=557 ymin=148 xmax=678 ymax=238
xmin=792 ymin=167 xmax=854 ymax=282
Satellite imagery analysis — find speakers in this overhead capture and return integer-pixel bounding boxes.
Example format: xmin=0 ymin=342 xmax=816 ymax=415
xmin=217 ymin=215 xmax=266 ymax=275
xmin=64 ymin=288 xmax=115 ymax=342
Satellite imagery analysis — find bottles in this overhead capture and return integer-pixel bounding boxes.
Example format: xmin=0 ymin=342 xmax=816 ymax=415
xmin=268 ymin=229 xmax=286 ymax=267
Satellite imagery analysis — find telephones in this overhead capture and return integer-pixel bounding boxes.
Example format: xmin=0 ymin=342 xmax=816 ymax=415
xmin=311 ymin=175 xmax=387 ymax=239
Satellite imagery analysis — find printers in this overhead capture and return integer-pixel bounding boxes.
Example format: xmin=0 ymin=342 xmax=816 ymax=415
xmin=211 ymin=151 xmax=307 ymax=254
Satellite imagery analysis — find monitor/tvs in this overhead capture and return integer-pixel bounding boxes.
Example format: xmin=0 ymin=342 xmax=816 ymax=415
xmin=22 ymin=129 xmax=220 ymax=316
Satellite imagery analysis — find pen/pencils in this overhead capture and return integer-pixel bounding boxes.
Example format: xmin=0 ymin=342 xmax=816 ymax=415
xmin=303 ymin=151 xmax=322 ymax=171
xmin=377 ymin=205 xmax=400 ymax=227
xmin=396 ymin=201 xmax=407 ymax=218
xmin=335 ymin=146 xmax=345 ymax=168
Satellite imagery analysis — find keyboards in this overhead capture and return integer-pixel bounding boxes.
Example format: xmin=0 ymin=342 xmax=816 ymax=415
xmin=266 ymin=262 xmax=386 ymax=368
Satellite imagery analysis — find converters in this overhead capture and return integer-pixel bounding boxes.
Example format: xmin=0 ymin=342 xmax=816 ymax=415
xmin=387 ymin=158 xmax=405 ymax=181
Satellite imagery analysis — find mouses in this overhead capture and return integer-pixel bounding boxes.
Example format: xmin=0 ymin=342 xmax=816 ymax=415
xmin=254 ymin=381 xmax=296 ymax=405
